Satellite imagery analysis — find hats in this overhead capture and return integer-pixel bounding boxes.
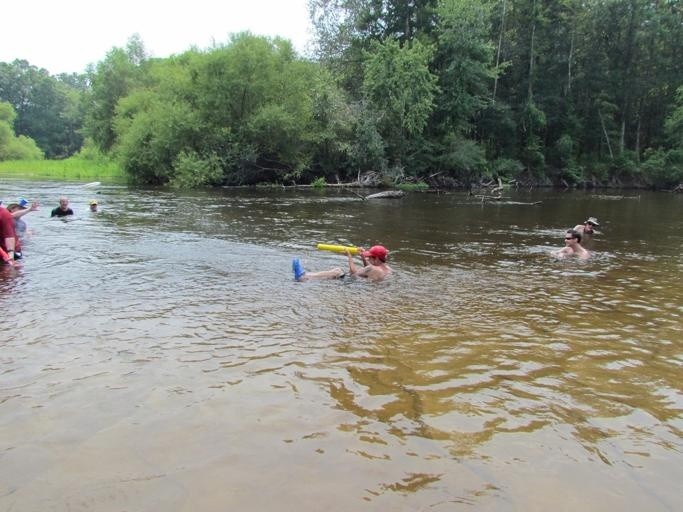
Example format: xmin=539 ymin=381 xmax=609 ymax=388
xmin=362 ymin=246 xmax=390 ymax=261
xmin=90 ymin=199 xmax=97 ymax=205
xmin=584 ymin=217 xmax=599 ymax=226
xmin=7 ymin=204 xmax=27 ymax=212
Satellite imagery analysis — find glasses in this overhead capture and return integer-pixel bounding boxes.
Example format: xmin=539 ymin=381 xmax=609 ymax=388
xmin=565 ymin=238 xmax=573 ymax=240
xmin=588 ymin=223 xmax=595 ymax=226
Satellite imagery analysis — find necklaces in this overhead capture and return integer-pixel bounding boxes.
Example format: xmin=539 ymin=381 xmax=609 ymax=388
xmin=582 ymin=228 xmax=594 ymax=235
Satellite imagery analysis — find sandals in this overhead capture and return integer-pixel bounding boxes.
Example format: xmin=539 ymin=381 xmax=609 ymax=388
xmin=292 ymin=258 xmax=305 ymax=280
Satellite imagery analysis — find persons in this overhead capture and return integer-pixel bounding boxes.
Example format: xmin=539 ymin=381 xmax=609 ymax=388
xmin=88 ymin=198 xmax=98 ymax=212
xmin=50 ymin=196 xmax=74 ymax=218
xmin=293 ymin=244 xmax=391 ymax=283
xmin=571 ymin=216 xmax=602 ymax=235
xmin=5 ymin=199 xmax=41 ymax=233
xmin=0 ymin=199 xmax=22 ymax=270
xmin=549 ymin=228 xmax=593 ymax=260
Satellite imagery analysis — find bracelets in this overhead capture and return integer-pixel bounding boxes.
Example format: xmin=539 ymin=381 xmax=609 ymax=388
xmin=7 ymin=249 xmax=16 ymax=253
